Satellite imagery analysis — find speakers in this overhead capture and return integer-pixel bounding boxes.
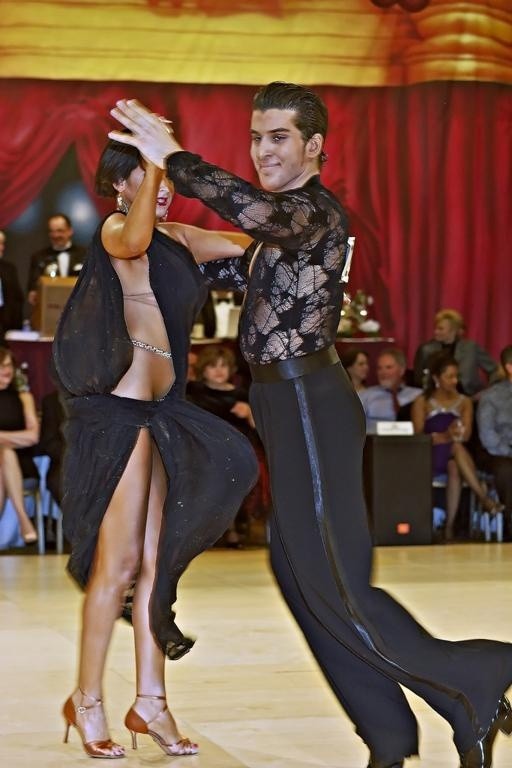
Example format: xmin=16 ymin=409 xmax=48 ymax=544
xmin=363 ymin=434 xmax=433 ymax=546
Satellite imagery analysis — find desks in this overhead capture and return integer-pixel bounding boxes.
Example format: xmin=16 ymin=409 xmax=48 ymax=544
xmin=0 ymin=332 xmax=396 ymax=422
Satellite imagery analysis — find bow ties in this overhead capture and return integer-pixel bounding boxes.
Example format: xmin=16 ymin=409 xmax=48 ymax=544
xmin=57 ymin=248 xmax=70 ymax=256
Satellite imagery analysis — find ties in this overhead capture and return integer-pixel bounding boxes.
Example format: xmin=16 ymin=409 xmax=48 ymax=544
xmin=388 ymin=387 xmax=401 ymax=421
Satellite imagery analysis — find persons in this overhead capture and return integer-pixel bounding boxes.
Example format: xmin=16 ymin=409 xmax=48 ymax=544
xmin=40 ymin=388 xmax=63 ymax=509
xmin=54 ymin=118 xmax=262 ymax=761
xmin=1 ymin=230 xmax=22 ymax=344
xmin=344 ymin=309 xmax=512 ymax=544
xmin=108 ymin=79 xmax=512 ymax=768
xmin=185 ymin=347 xmax=258 ymax=549
xmin=1 ymin=345 xmax=41 ymax=542
xmin=199 ymin=288 xmax=244 ymax=338
xmin=26 ymin=212 xmax=88 ymax=306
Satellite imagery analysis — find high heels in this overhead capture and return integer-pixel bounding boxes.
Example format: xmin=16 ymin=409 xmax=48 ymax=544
xmin=125 ymin=694 xmax=199 ymax=757
xmin=63 ymin=687 xmax=126 ymax=760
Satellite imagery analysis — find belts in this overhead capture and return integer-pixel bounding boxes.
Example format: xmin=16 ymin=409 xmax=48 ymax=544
xmin=249 ymin=345 xmax=340 ymax=385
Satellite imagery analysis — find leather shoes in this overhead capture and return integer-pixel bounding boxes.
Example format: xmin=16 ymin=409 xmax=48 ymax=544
xmin=19 ymin=524 xmax=40 ymax=543
xmin=459 ymin=693 xmax=512 ymax=768
xmin=224 ymin=528 xmax=244 ymax=549
